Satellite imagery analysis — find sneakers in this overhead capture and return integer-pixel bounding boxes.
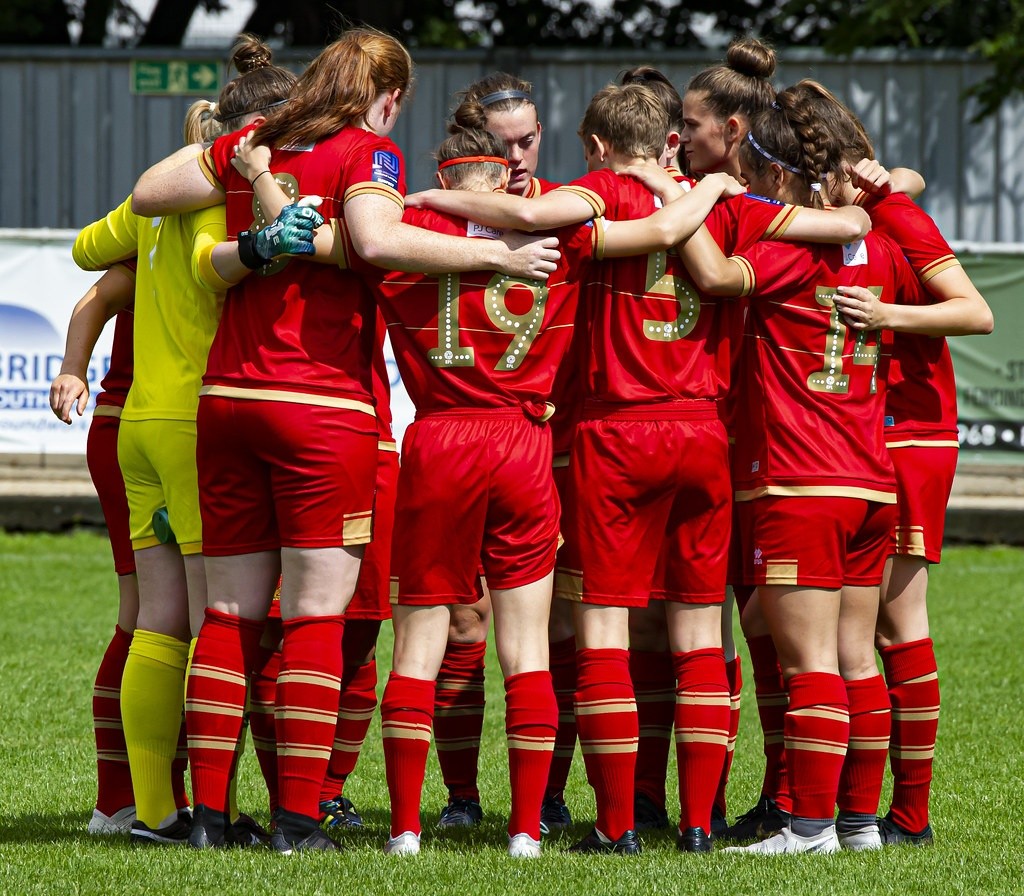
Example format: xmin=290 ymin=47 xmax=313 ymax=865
xmin=269 ymin=823 xmax=346 ymax=856
xmin=566 ymin=827 xmax=642 ymax=854
xmin=130 ymin=814 xmax=189 ymax=846
xmin=729 ymin=803 xmax=789 ymax=839
xmin=386 ymin=828 xmax=423 ymax=855
xmin=504 ymin=830 xmax=543 ymax=858
xmin=87 ymin=805 xmax=137 ymax=834
xmin=836 ymin=823 xmax=882 ymax=849
xmin=189 ymin=807 xmax=270 ymax=846
xmin=438 ymin=802 xmax=482 ymax=831
xmin=540 ymin=801 xmax=573 ymax=834
xmin=720 ymin=825 xmax=842 ymax=855
xmin=677 ymin=827 xmax=713 ymax=851
xmin=635 ymin=808 xmax=670 ymax=829
xmin=320 ymin=797 xmax=370 ymax=833
xmin=877 ymin=813 xmax=933 ymax=844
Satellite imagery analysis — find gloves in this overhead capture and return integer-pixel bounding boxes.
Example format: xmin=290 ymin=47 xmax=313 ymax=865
xmin=241 ymin=196 xmax=324 ymax=275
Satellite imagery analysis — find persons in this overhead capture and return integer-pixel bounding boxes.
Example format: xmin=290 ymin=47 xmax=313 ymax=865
xmin=230 ymin=98 xmax=751 ymax=859
xmin=130 ymin=26 xmax=563 ymax=861
xmin=404 ymin=35 xmax=996 ymax=858
xmin=70 ymin=36 xmax=324 ymax=845
xmin=247 ymin=303 xmax=400 ymax=832
xmin=49 ymin=100 xmax=224 ymax=833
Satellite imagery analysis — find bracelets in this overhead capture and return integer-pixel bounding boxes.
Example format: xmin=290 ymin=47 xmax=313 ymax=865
xmin=251 ymin=170 xmax=272 ymax=189
